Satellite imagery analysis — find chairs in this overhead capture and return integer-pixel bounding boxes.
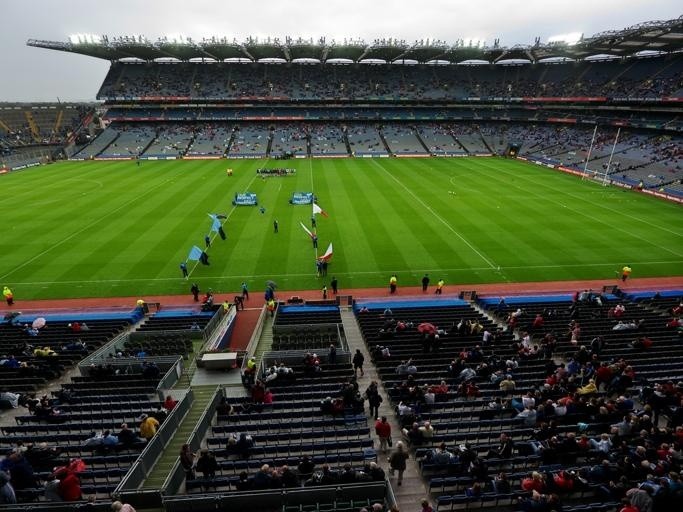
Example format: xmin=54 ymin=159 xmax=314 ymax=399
xmin=187 ymin=296 xmax=683 ymax=511
xmin=0 ymin=312 xmax=210 ymax=511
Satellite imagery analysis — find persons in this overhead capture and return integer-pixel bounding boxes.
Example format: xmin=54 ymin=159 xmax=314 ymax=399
xmin=1 ymin=105 xmax=104 ymax=174
xmin=316 ymin=259 xmax=328 ymax=277
xmin=530 ymin=64 xmax=682 ymax=196
xmin=0 ymin=274 xmax=683 ymax=512
xmin=256 ymin=167 xmax=296 ymax=181
xmin=259 ymin=205 xmax=265 ymax=214
xmin=232 ymin=192 xmax=238 ymax=206
xmin=311 ymin=218 xmax=318 ymax=248
xmin=227 ymin=167 xmax=232 ymax=176
xmin=274 ymin=218 xmax=279 ymax=232
xmin=101 ymin=65 xmax=556 ymax=161
xmin=205 ymin=235 xmax=211 ymax=248
xmin=180 ymin=262 xmax=189 ymax=277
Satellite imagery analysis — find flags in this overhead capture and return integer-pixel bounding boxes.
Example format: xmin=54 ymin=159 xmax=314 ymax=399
xmin=292 ymin=192 xmax=313 ymax=205
xmin=312 ymin=203 xmax=328 ymax=217
xmin=235 ymin=193 xmax=256 ymax=205
xmin=300 ymin=222 xmax=313 ymax=239
xmin=318 ymin=241 xmax=332 ymax=260
xmin=208 ymin=213 xmax=228 ymax=241
xmin=188 ymin=246 xmax=210 ymax=266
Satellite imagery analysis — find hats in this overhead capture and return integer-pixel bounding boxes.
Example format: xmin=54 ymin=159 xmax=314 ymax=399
xmin=53 ymin=467 xmax=67 ymax=475
xmin=369 ymin=462 xmax=377 ymax=468
xmin=420 ymin=498 xmax=427 ymax=505
xmin=440 ymin=443 xmax=446 ymax=447
xmin=372 ymin=503 xmax=382 ymax=510
xmin=138 ymin=414 xmax=148 ymax=421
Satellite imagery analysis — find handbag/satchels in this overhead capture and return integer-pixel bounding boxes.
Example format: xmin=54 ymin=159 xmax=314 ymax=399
xmin=386 ymin=436 xmax=392 ymax=447
